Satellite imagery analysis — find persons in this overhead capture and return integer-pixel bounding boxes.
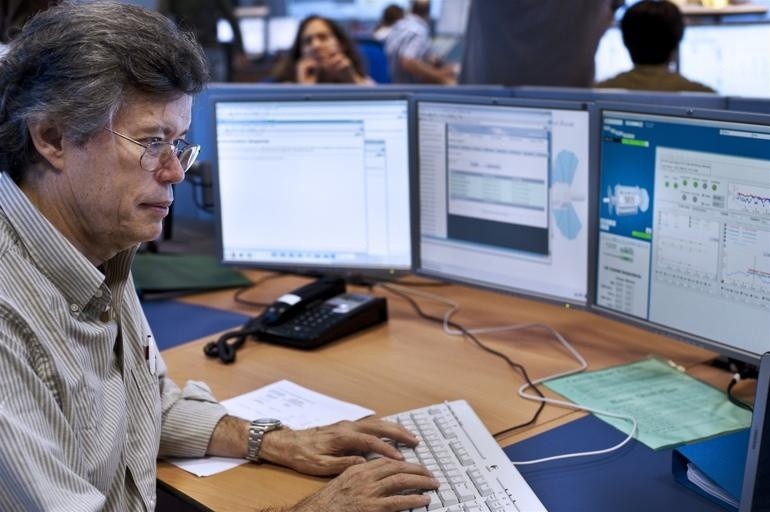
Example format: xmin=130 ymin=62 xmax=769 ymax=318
xmin=0 ymin=1 xmax=440 ymax=512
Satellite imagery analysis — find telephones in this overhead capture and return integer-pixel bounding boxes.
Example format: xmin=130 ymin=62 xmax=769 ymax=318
xmin=244 ymin=275 xmax=388 ymax=350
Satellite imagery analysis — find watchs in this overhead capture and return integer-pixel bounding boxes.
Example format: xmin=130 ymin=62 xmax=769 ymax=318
xmin=245 ymin=419 xmax=282 ymax=464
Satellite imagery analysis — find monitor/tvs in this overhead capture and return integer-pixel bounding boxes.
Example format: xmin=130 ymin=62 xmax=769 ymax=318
xmin=586 ymin=101 xmax=770 ymax=367
xmin=209 ymin=92 xmax=414 ymax=280
xmin=677 ymin=21 xmax=770 ymax=99
xmin=590 ymin=23 xmax=637 ymax=86
xmin=216 ymin=14 xmax=303 ymax=61
xmin=413 ymin=95 xmax=595 ymax=311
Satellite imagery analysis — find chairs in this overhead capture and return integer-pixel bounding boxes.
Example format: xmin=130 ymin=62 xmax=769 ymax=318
xmin=350 ymin=36 xmax=394 ymax=86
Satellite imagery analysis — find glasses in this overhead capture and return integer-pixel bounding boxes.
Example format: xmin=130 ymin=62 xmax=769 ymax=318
xmin=100 ymin=125 xmax=201 ymax=177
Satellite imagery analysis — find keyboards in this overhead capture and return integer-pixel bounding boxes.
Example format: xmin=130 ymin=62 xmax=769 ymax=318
xmin=363 ymin=400 xmax=548 ymax=512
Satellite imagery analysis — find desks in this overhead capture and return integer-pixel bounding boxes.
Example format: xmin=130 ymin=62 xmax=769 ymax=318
xmin=134 ymin=265 xmax=768 ymax=511
